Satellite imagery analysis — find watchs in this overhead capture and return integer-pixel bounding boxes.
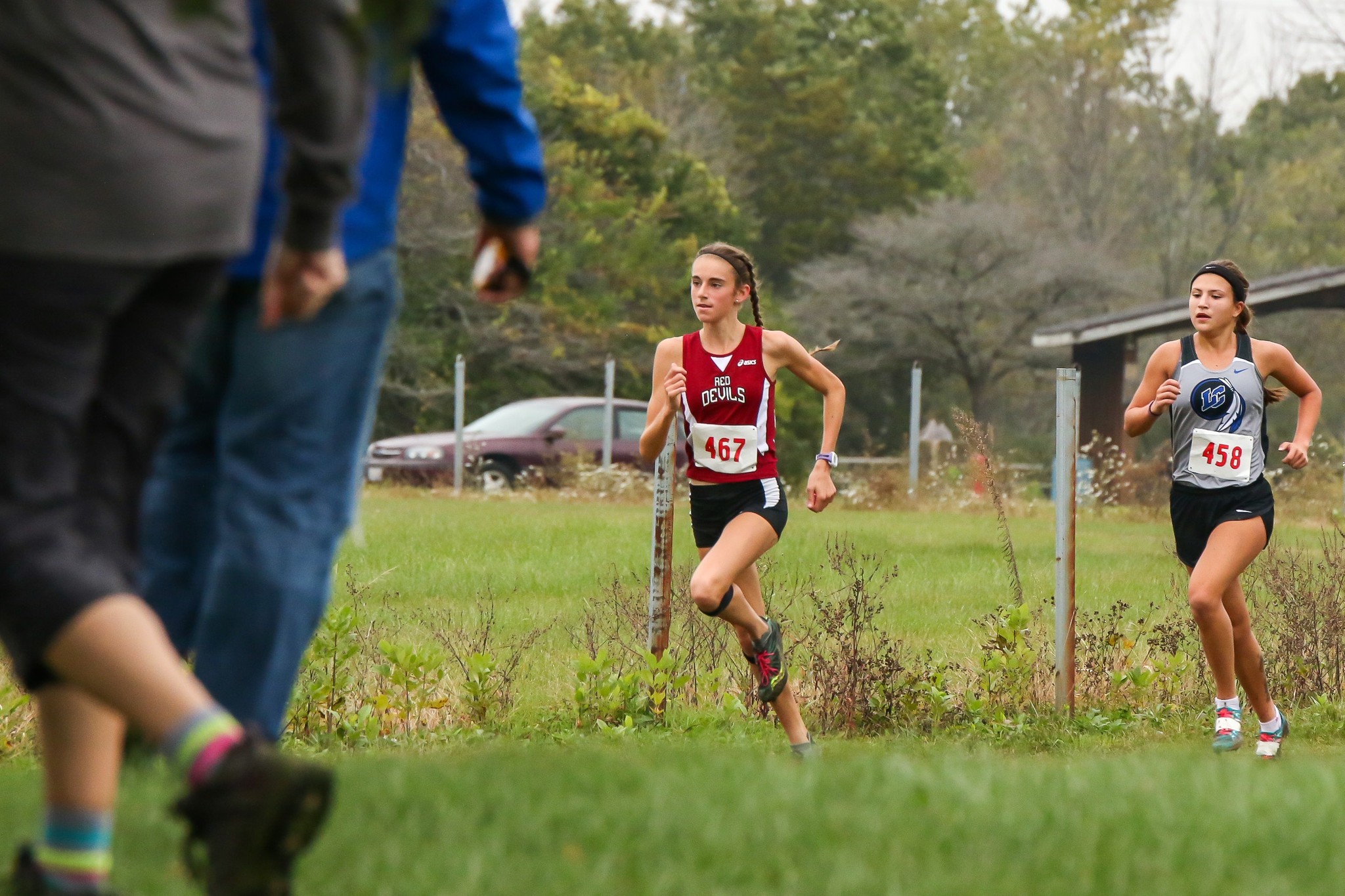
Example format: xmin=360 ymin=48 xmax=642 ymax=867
xmin=815 ymin=451 xmax=838 ymax=468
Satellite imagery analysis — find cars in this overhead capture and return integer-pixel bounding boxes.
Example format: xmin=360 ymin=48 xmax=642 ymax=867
xmin=364 ymin=396 xmax=687 ymax=491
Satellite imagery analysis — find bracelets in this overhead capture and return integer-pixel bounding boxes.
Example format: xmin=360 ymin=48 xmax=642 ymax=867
xmin=1148 ymin=400 xmax=1163 ymax=417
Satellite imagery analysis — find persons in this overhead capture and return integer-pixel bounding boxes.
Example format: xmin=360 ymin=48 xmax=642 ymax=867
xmin=1 ymin=0 xmax=554 ymax=896
xmin=1122 ymin=259 xmax=1323 ymax=763
xmin=638 ymin=242 xmax=847 ymax=762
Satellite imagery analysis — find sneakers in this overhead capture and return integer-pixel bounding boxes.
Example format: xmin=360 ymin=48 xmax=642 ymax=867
xmin=167 ymin=719 xmax=336 ymax=895
xmin=1211 ymin=706 xmax=1244 ymax=753
xmin=785 ymin=733 xmax=820 ymax=759
xmin=750 ymin=614 xmax=788 ymax=704
xmin=0 ymin=839 xmax=121 ymax=895
xmin=1255 ymin=711 xmax=1289 ymax=761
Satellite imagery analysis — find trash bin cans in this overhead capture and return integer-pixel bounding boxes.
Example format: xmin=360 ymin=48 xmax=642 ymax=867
xmin=1050 ymin=454 xmax=1093 ymax=503
